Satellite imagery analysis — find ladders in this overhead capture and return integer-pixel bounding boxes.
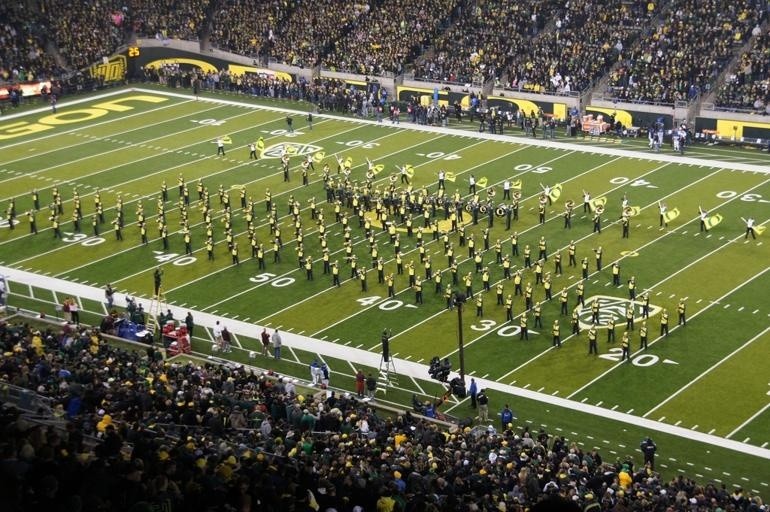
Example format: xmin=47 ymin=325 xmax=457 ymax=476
xmin=145 ymin=285 xmax=168 ymax=336
xmin=373 ymin=351 xmax=401 ymax=397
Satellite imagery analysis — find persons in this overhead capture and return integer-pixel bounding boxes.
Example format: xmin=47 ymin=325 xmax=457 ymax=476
xmin=3 ymin=153 xmax=757 ymax=362
xmin=0 ymin=358 xmax=770 ymax=511
xmin=0 ymin=0 xmax=770 ymax=157
